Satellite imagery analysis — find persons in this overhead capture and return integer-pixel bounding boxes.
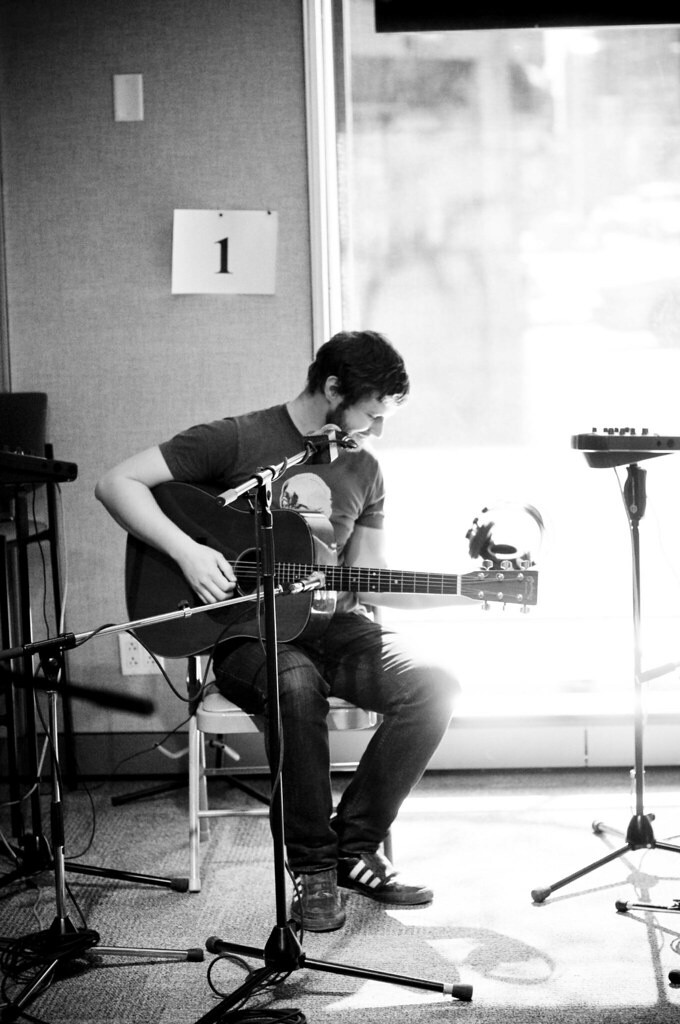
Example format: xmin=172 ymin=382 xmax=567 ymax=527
xmin=95 ymin=331 xmax=487 ymax=932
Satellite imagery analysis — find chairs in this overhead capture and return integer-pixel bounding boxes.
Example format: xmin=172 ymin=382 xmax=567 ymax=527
xmin=188 ymin=655 xmax=394 ymax=891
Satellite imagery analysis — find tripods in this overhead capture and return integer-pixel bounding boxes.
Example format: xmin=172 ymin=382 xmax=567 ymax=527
xmin=529 ymin=457 xmax=680 ymax=904
xmin=0 ymin=434 xmax=475 ymax=1024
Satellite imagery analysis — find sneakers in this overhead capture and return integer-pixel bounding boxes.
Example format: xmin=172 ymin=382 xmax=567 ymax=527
xmin=339 ymin=852 xmax=434 ymax=905
xmin=290 ymin=866 xmax=346 ymax=931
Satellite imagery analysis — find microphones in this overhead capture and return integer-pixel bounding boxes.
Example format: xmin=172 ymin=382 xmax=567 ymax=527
xmin=321 ymin=424 xmax=358 ymax=449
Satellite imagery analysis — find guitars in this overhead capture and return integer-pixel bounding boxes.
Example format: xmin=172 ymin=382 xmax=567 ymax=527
xmin=124 ymin=480 xmax=539 ymax=660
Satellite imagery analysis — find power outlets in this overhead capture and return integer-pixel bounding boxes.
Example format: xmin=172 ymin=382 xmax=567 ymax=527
xmin=119 ymin=632 xmax=165 ymax=676
